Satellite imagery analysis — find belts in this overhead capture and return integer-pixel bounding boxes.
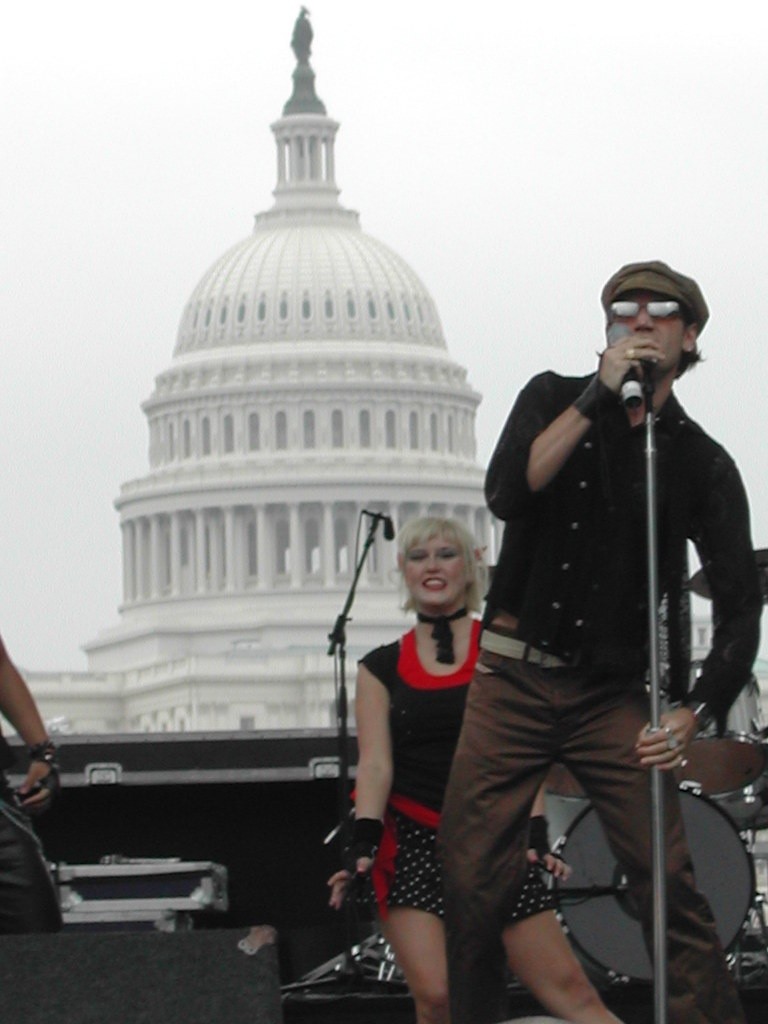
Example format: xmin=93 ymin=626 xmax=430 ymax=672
xmin=478 ymin=630 xmax=566 ymax=668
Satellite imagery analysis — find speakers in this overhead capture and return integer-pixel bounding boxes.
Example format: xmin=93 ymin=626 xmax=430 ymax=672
xmin=0 ymin=924 xmax=284 ymax=1024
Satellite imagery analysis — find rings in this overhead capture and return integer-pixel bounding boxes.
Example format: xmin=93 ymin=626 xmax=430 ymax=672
xmin=667 ymin=737 xmax=679 ymax=749
xmin=664 ymin=726 xmax=673 ymax=734
xmin=625 ymin=347 xmax=634 ymax=361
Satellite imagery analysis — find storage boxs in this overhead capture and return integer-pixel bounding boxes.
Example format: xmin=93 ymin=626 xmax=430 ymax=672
xmin=53 ymin=852 xmax=227 ymax=933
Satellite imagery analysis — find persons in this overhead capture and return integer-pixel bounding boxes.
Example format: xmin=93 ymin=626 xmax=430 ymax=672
xmin=328 ymin=516 xmax=631 ymax=1024
xmin=0 ymin=637 xmax=62 ymax=937
xmin=440 ymin=260 xmax=762 ymax=1023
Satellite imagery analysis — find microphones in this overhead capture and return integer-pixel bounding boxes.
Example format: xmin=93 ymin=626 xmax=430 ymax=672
xmin=606 ymin=322 xmax=646 ymax=411
xmin=360 ymin=510 xmax=395 ymax=540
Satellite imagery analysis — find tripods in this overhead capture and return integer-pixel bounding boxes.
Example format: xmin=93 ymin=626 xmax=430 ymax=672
xmin=281 ymin=517 xmax=407 ymax=993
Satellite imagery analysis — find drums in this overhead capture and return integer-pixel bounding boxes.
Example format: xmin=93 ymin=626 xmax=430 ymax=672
xmin=661 ymin=656 xmax=768 ymax=831
xmin=550 ymin=786 xmax=755 ymax=984
xmin=545 ymin=763 xmax=589 ymax=800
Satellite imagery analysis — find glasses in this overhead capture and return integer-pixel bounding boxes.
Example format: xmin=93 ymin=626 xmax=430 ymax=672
xmin=611 ymin=299 xmax=681 ymax=322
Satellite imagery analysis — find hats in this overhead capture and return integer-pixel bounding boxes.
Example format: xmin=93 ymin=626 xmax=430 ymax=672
xmin=601 ymin=261 xmax=709 ymax=337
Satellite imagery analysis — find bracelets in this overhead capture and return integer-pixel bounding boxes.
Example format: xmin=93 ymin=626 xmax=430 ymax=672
xmin=572 ymin=373 xmax=619 ymax=424
